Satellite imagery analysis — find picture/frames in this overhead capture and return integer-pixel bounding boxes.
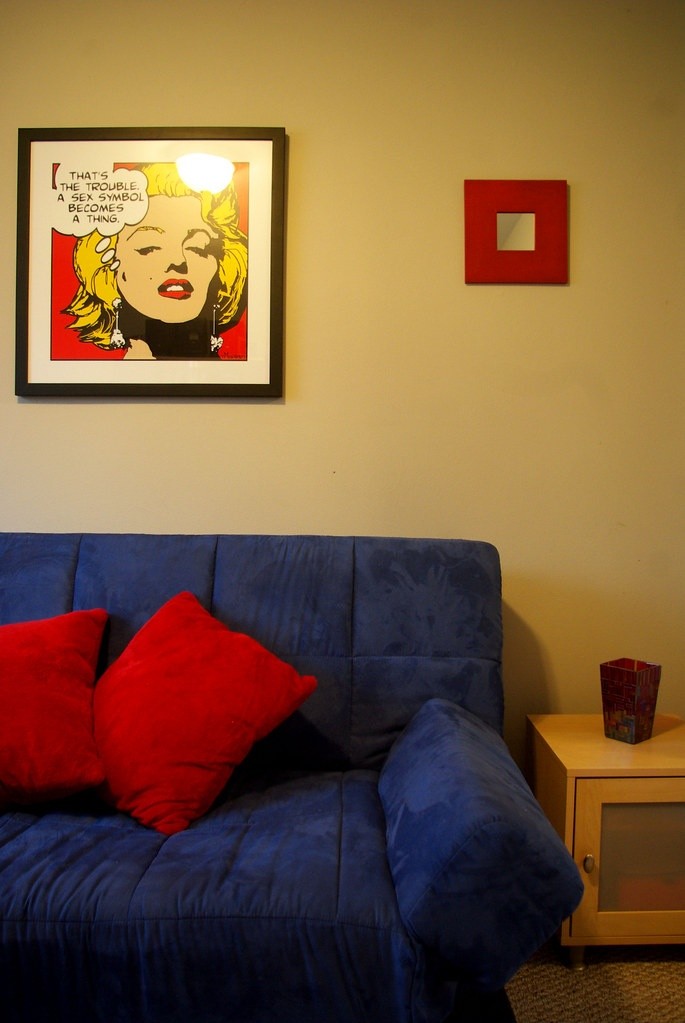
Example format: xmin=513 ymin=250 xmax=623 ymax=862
xmin=16 ymin=128 xmax=284 ymax=397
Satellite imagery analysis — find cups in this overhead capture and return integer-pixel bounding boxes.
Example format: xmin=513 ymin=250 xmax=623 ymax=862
xmin=599 ymin=658 xmax=662 ymax=745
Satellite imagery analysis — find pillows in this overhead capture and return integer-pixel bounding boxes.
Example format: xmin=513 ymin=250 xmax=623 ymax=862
xmin=91 ymin=590 xmax=319 ymax=835
xmin=0 ymin=608 xmax=111 ymax=800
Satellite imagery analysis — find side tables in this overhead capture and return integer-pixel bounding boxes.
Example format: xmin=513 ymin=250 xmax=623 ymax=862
xmin=527 ymin=709 xmax=684 ymax=970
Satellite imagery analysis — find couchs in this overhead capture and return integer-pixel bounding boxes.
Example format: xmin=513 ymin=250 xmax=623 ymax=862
xmin=0 ymin=529 xmax=583 ymax=1022
